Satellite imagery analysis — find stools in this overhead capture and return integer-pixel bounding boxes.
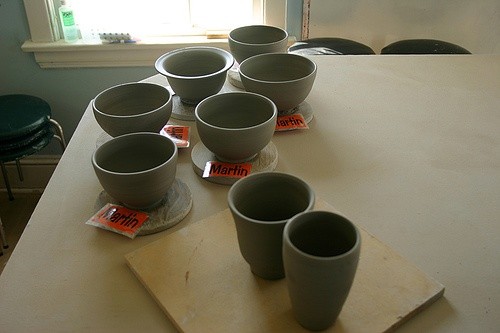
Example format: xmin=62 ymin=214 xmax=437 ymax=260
xmin=288 ymin=37 xmax=375 ymax=54
xmin=380 ymin=39 xmax=471 ymax=55
xmin=0 ymin=95 xmax=67 ymax=257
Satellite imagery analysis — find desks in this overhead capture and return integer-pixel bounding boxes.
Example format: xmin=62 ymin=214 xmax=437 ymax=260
xmin=0 ymin=54 xmax=500 ymax=333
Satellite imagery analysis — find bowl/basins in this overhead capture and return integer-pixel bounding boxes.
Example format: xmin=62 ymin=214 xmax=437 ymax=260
xmin=156 ymin=46 xmax=234 ymax=105
xmin=195 ymin=90 xmax=278 ymax=162
xmin=228 ymin=24 xmax=288 ymax=64
xmin=238 ymin=51 xmax=317 ymax=110
xmin=92 ymin=82 xmax=173 ymax=137
xmin=92 ymin=132 xmax=179 ymax=213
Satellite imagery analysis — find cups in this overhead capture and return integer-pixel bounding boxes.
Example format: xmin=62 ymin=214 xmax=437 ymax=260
xmin=282 ymin=208 xmax=363 ymax=329
xmin=226 ymin=171 xmax=317 ymax=279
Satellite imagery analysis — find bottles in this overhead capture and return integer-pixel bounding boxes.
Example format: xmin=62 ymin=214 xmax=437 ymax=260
xmin=58 ymin=0 xmax=78 ymax=42
